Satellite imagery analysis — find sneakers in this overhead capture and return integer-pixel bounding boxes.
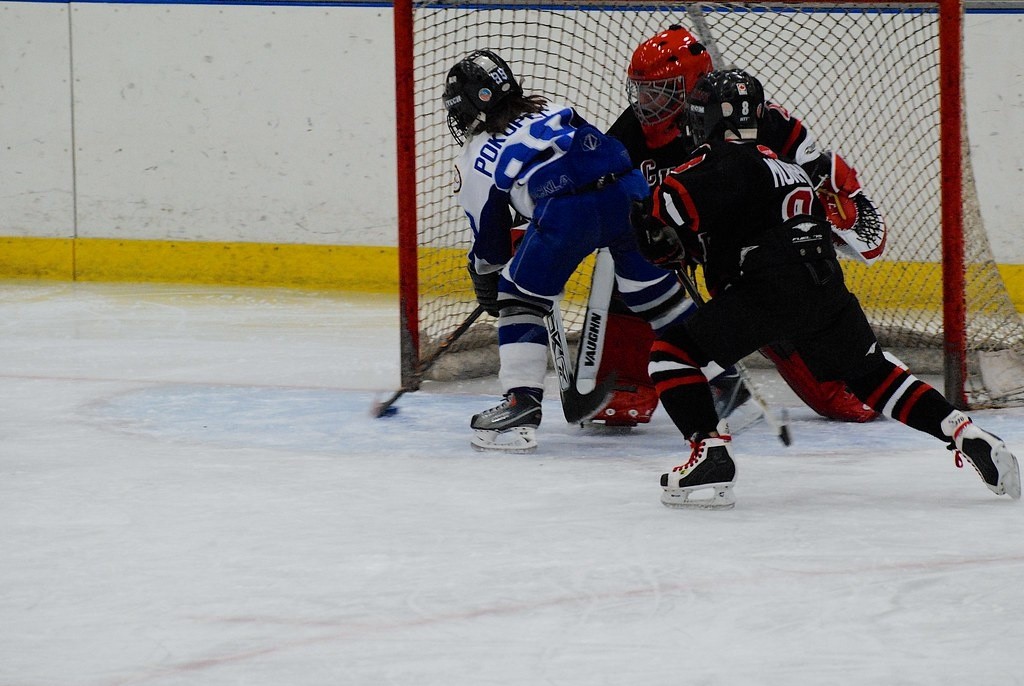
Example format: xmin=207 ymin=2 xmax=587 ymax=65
xmin=471 ymin=387 xmax=544 ymax=453
xmin=706 ymin=366 xmax=763 ymax=439
xmin=660 ymin=418 xmax=737 ymax=510
xmin=941 ymin=408 xmax=1022 ymax=499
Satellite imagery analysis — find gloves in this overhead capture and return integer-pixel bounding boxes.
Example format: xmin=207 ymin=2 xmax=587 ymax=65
xmin=466 ymin=262 xmax=501 ymax=318
xmin=630 ymin=212 xmax=684 ymax=271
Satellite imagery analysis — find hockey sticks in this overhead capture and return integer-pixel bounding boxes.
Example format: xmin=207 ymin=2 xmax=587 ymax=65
xmin=675 ymin=264 xmax=794 ymax=450
xmin=364 ymin=301 xmax=486 ymax=421
xmin=686 ymin=3 xmax=869 ymax=264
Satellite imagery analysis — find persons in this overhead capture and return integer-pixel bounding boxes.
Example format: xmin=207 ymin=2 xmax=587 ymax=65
xmin=571 ymin=25 xmax=888 ymax=436
xmin=629 ymin=67 xmax=1023 ymax=510
xmin=442 ymin=51 xmax=767 ymax=454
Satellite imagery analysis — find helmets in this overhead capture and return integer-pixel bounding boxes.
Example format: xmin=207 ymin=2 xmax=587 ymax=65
xmin=688 ymin=69 xmax=764 ymax=145
xmin=441 ymin=50 xmax=518 ymax=126
xmin=628 ymin=24 xmax=714 ymax=148
